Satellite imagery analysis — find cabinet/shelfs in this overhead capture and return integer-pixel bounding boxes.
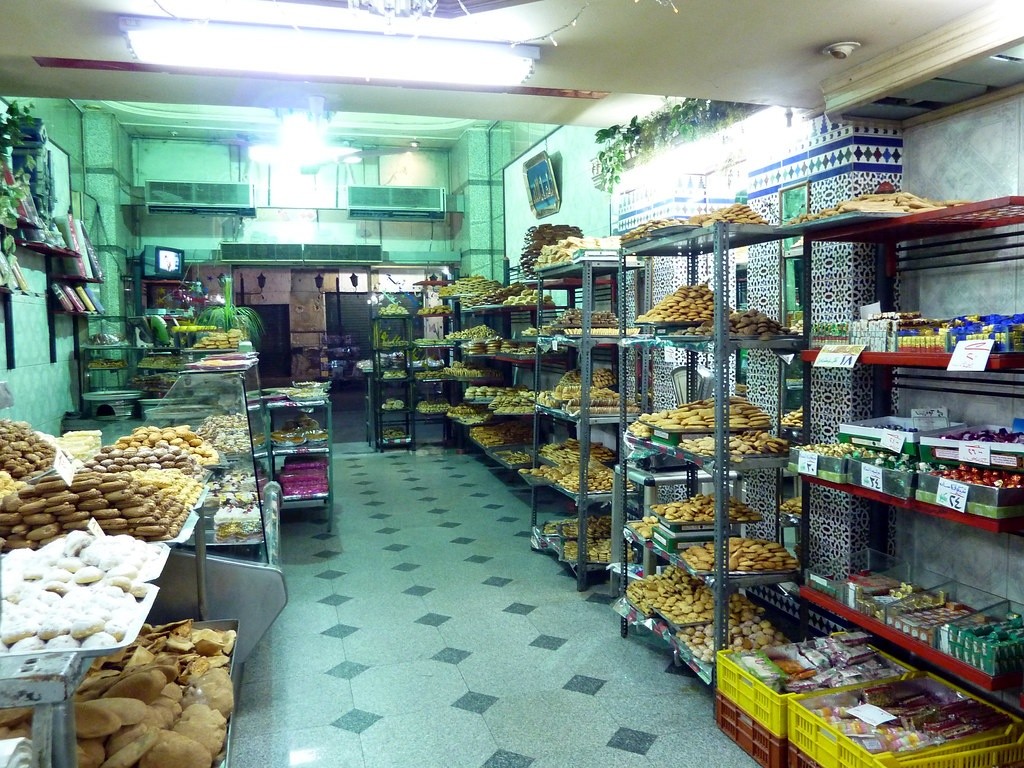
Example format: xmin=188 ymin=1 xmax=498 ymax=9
xmin=365 ymin=193 xmax=1023 ymax=714
xmin=0 ymin=209 xmax=106 ymax=370
xmin=265 ymin=390 xmax=334 ymax=533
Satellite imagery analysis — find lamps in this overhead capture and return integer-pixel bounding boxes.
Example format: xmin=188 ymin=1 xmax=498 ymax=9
xmin=243 ymin=272 xmax=266 ymax=295
xmin=314 ymin=272 xmax=327 ymax=294
xmin=350 ymin=272 xmax=358 ymax=293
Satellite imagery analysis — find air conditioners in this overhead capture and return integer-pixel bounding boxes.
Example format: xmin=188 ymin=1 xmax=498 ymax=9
xmin=345 ymin=184 xmax=446 ymax=223
xmin=303 ymin=243 xmax=384 ymax=265
xmin=219 ymin=244 xmax=303 ymax=265
xmin=144 ymin=178 xmax=257 ymax=219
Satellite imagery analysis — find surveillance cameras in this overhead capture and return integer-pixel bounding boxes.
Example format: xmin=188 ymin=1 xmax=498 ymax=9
xmin=829 ymin=46 xmax=853 ymax=59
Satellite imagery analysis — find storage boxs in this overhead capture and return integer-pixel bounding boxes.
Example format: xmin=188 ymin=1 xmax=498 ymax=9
xmin=714 ymin=416 xmax=1024 ymax=767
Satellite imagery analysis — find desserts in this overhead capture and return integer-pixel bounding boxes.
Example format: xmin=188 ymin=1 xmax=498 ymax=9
xmin=203 ymin=471 xmax=261 ymax=538
xmin=270 ymin=426 xmax=329 ymax=447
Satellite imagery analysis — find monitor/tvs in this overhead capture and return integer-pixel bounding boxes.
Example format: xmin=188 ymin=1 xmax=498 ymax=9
xmin=142 ymin=245 xmax=185 ymax=281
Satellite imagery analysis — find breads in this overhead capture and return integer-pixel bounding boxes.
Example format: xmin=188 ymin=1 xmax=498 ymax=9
xmin=0 ymin=529 xmax=160 ymax=653
xmin=0 ymin=661 xmax=234 ymax=768
xmin=620 ymin=202 xmax=769 ymax=246
xmin=783 ymin=192 xmax=966 ymax=214
xmin=628 ymin=491 xmax=801 ymax=666
xmin=628 ymin=396 xmax=803 ymax=455
xmin=377 ymin=224 xmax=633 ymax=562
xmin=0 ymin=417 xmax=211 ymax=548
xmin=193 ymin=328 xmax=243 ymax=349
xmin=635 ymin=283 xmax=799 ymax=343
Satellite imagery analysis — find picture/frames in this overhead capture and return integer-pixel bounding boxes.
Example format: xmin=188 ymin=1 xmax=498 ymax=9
xmin=522 ymin=150 xmax=561 ymax=220
